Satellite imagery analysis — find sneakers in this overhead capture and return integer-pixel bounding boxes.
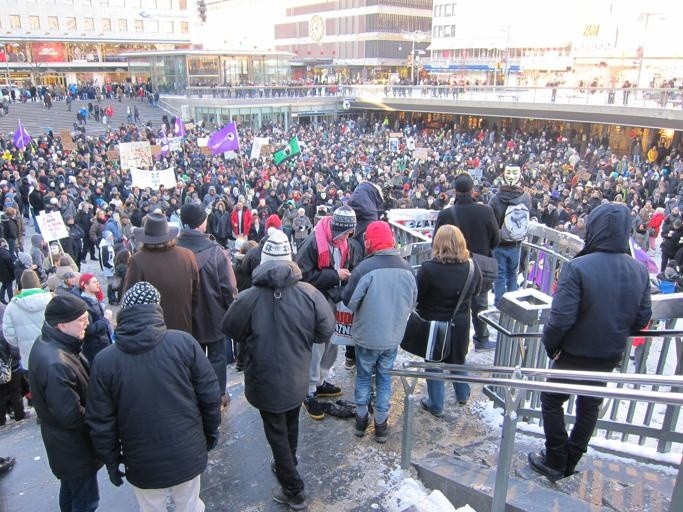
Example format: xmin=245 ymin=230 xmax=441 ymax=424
xmin=420 ymin=396 xmax=445 ymax=416
xmin=269 ymin=457 xmax=278 ymax=475
xmin=271 ymin=484 xmax=308 ymax=509
xmin=318 ymin=380 xmax=342 ymax=397
xmin=303 ymin=392 xmax=325 ymax=420
xmin=476 ymin=340 xmax=494 ymax=350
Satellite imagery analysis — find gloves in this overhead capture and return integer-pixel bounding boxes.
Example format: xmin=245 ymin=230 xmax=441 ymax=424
xmin=205 ymin=431 xmax=220 ymax=451
xmin=105 ymin=453 xmax=126 ymax=487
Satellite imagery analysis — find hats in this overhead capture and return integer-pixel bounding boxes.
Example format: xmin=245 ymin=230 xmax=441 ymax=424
xmin=363 ymin=222 xmax=394 ymax=254
xmin=122 ymin=281 xmax=161 ymax=309
xmin=78 ymin=273 xmax=94 ymax=289
xmin=61 ymin=271 xmax=73 ymax=278
xmin=45 ymin=293 xmax=87 ymax=328
xmin=132 ymin=214 xmax=180 ymax=244
xmin=102 ymin=230 xmax=114 ymax=242
xmin=19 ymin=268 xmax=41 ymax=290
xmin=181 ymin=204 xmax=207 ymax=231
xmin=260 ymin=231 xmax=291 ymax=264
xmin=456 ymin=174 xmax=473 ymax=191
xmin=331 ymin=205 xmax=357 ymax=233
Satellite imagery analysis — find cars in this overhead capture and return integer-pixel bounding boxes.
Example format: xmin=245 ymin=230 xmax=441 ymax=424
xmin=642 ymin=84 xmax=677 ymax=101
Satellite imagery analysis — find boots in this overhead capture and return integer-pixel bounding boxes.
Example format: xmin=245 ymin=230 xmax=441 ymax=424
xmin=354 ymin=412 xmax=372 ymax=438
xmin=373 ymin=418 xmax=390 ymax=443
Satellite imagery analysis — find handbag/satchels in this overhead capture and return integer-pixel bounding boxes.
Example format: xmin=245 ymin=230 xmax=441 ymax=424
xmin=329 ymin=281 xmax=356 ymax=348
xmin=0 ymin=356 xmax=12 ymax=385
xmin=471 ymin=250 xmax=498 ymax=285
xmin=400 ymin=311 xmax=453 ymax=363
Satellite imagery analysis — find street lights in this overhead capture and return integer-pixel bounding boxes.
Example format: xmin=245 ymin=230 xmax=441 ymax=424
xmin=0 ymin=42 xmax=18 ymax=104
xmin=635 ymin=13 xmax=665 ymax=90
xmin=397 ymin=28 xmax=425 ymax=82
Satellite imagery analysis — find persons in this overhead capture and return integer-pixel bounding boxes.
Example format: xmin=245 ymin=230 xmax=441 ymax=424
xmin=216 ymin=226 xmax=336 ymax=510
xmin=528 ymin=203 xmax=652 ymax=483
xmin=340 ymin=220 xmax=417 ymax=442
xmin=0 ymin=455 xmax=15 ymax=473
xmin=83 ymin=280 xmax=221 ymax=512
xmin=0 ymin=69 xmax=683 ymax=425
xmin=27 ymin=294 xmax=105 ymax=511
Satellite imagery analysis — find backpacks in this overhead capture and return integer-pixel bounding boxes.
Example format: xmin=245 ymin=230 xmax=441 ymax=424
xmin=500 ymin=202 xmax=529 ymax=243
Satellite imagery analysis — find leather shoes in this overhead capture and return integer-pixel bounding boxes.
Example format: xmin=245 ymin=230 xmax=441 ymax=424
xmin=528 ymin=452 xmax=565 ymax=483
xmin=540 ymin=449 xmax=576 ymax=476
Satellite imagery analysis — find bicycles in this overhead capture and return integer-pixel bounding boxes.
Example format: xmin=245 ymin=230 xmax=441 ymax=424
xmin=40 ymin=99 xmax=49 ymax=111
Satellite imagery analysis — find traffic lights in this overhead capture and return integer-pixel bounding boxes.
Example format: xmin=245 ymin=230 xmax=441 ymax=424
xmin=196 ymin=0 xmax=206 ymax=22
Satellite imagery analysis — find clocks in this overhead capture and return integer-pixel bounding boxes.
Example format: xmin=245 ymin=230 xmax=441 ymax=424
xmin=307 ymin=14 xmax=325 ymax=43
